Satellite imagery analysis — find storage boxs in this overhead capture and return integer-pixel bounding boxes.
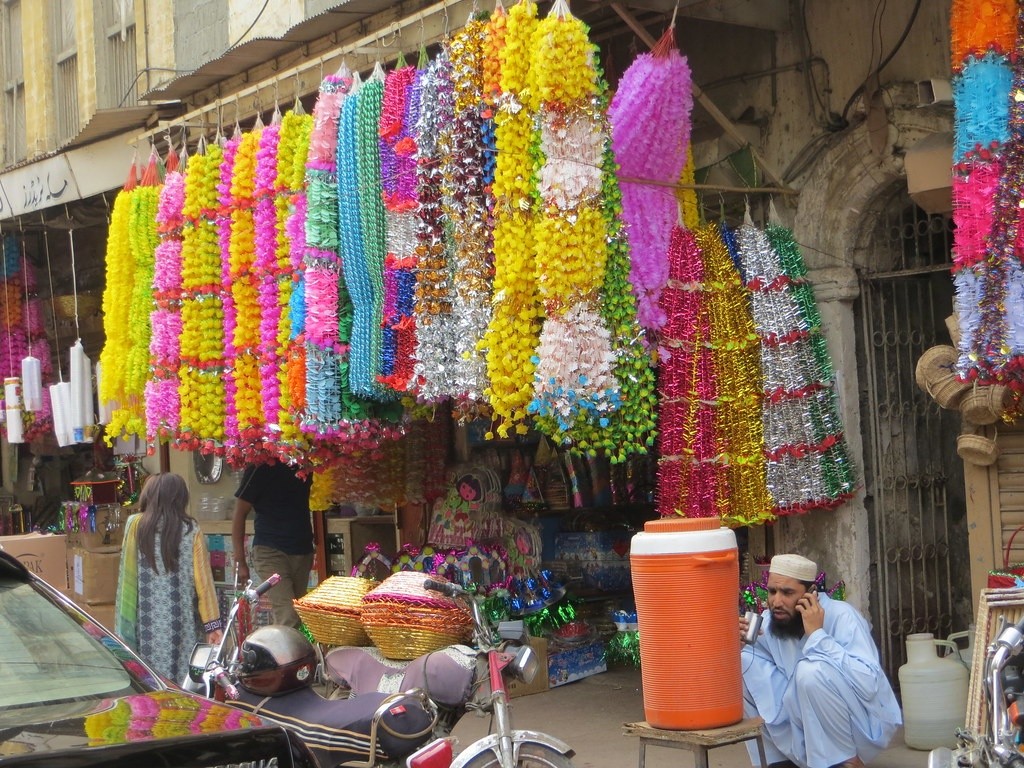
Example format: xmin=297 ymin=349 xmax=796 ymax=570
xmin=548 ymin=641 xmax=607 ymax=688
xmin=62 ymin=502 xmax=140 ymax=553
xmin=507 ymin=636 xmax=547 ymax=699
xmin=66 ymin=547 xmax=122 ymax=605
xmin=0 ymin=530 xmax=67 ymax=590
xmin=80 ymin=606 xmax=115 ymax=634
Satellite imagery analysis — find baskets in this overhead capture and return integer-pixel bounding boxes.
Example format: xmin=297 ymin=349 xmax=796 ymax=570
xmin=362 ymin=571 xmax=473 ymax=660
xmin=957 ymin=424 xmax=1002 ymax=465
xmin=292 ymin=576 xmax=381 ymax=647
xmin=916 ymin=310 xmax=1024 ymax=426
xmin=988 ymin=525 xmax=1024 ymax=588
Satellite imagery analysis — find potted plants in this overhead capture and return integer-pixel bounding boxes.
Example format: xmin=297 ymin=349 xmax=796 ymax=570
xmin=71 ymin=468 xmax=121 ymax=505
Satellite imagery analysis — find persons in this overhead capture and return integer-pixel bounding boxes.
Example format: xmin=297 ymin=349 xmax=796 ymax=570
xmin=116 ymin=473 xmax=225 ymax=697
xmin=739 ymin=553 xmax=903 ymax=768
xmin=231 ymin=460 xmax=316 ymax=631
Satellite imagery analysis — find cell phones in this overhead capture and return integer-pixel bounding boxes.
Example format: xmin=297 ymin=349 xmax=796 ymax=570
xmin=796 ymin=584 xmax=818 ymax=614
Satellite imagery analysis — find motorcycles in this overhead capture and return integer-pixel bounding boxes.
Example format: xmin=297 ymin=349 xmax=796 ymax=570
xmin=927 ymin=611 xmax=1024 ymax=768
xmin=178 ymin=560 xmax=459 ymax=768
xmin=312 ymin=580 xmax=578 ymax=768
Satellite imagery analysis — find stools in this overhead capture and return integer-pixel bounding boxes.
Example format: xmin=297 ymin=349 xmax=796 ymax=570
xmin=621 ymin=717 xmax=768 ymax=768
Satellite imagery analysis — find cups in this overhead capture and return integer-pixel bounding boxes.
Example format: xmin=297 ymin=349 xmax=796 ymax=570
xmin=741 ymin=612 xmax=763 ymax=644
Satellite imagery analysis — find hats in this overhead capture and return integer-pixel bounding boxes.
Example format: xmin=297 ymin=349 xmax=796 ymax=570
xmin=769 ymin=554 xmax=817 ymax=581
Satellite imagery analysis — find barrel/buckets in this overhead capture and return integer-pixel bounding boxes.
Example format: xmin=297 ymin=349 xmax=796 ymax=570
xmin=629 ymin=516 xmax=743 ymax=730
xmin=898 ymin=633 xmax=969 ymax=750
xmin=944 ymin=620 xmax=976 ymax=671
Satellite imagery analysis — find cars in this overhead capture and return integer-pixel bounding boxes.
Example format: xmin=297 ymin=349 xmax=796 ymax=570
xmin=0 ymin=547 xmax=321 ymax=768
